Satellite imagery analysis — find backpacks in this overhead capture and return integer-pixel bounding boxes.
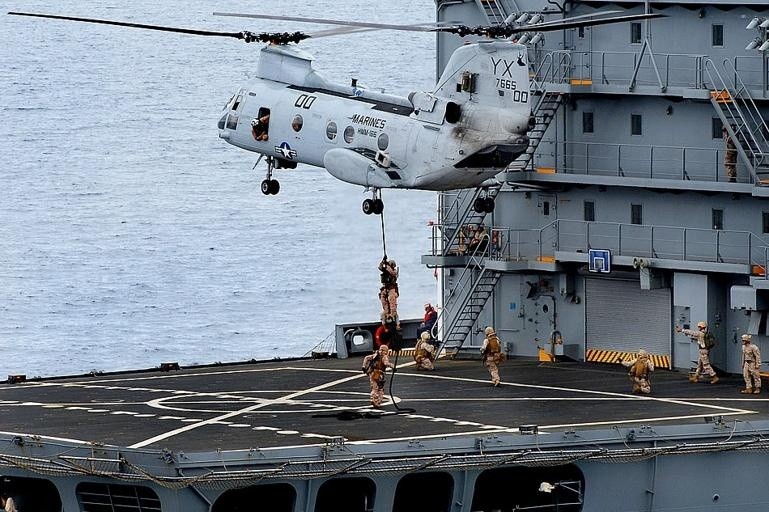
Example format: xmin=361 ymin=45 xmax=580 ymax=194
xmin=701 ymin=330 xmax=715 ymax=349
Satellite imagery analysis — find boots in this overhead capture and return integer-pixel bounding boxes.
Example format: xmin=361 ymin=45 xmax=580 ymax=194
xmin=710 ymin=376 xmax=719 ymax=384
xmin=741 ymin=387 xmax=753 ymax=394
xmin=753 ymin=387 xmax=760 ymax=394
xmin=633 ymin=383 xmax=641 ymax=394
xmin=689 ymin=376 xmax=699 ymax=382
xmin=494 ymin=379 xmax=500 ymax=387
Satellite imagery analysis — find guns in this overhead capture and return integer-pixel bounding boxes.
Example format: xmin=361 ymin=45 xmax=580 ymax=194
xmin=363 ymin=350 xmax=381 ymax=373
xmin=427 ymin=339 xmax=445 ymax=346
xmin=478 ymin=346 xmax=490 ymax=361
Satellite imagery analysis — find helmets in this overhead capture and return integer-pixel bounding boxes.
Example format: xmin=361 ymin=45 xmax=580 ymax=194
xmin=485 ymin=327 xmax=493 ymax=334
xmin=379 ymin=345 xmax=388 ymax=354
xmin=698 ymin=322 xmax=706 ymax=329
xmin=741 ymin=334 xmax=750 ymax=341
xmin=389 ymin=259 xmax=396 ymax=268
xmin=638 ymin=349 xmax=647 ymax=358
xmin=421 ymin=332 xmax=430 ymax=340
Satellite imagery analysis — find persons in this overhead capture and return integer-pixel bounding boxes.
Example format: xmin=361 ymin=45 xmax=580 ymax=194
xmin=360 ymin=346 xmax=396 ymax=409
xmin=420 ymin=303 xmax=439 ymax=341
xmin=739 ymin=334 xmax=762 ymax=394
xmin=250 ymin=114 xmax=269 ymax=142
xmin=480 ymin=327 xmax=505 ymax=388
xmin=616 ymin=349 xmax=655 ymax=393
xmin=413 ymin=335 xmax=438 ymax=371
xmin=674 ymin=320 xmax=720 ymax=383
xmin=379 ymin=255 xmax=402 ymax=334
xmin=458 ymin=224 xmax=501 ymax=257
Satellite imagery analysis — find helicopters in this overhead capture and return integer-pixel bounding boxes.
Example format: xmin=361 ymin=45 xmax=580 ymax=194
xmin=6 ymin=11 xmax=668 ymax=215
